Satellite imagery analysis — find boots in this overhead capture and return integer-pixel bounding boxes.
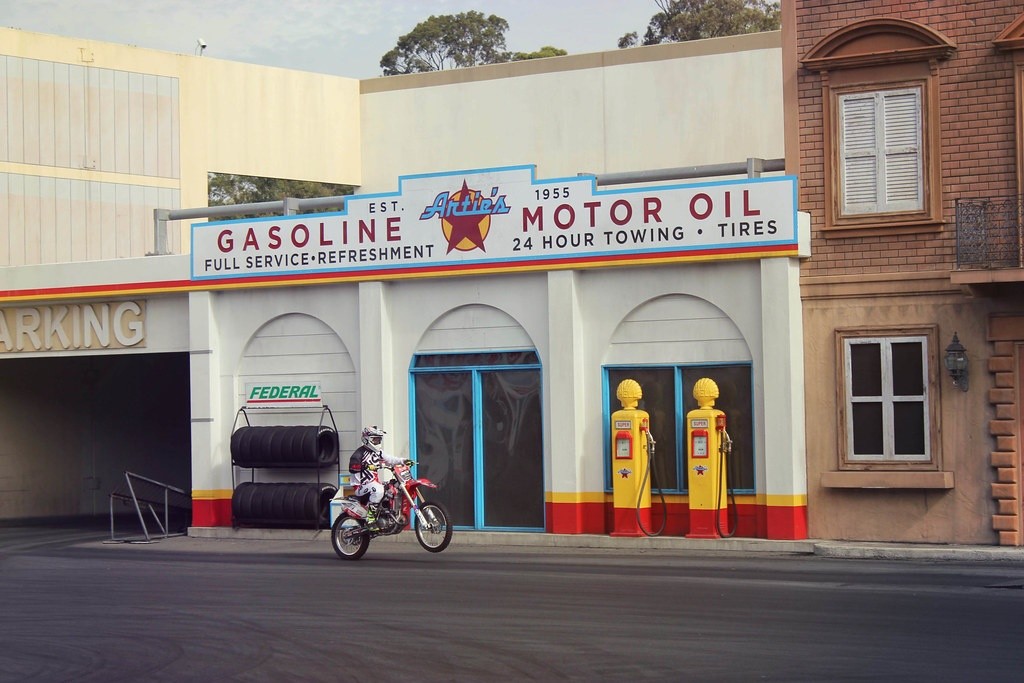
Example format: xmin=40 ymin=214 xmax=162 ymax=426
xmin=364 ymin=500 xmax=380 ymax=531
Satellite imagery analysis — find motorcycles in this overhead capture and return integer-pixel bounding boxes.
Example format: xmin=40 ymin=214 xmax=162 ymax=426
xmin=329 ymin=460 xmax=453 ymax=560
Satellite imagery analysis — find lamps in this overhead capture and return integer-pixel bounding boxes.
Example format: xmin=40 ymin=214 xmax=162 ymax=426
xmin=945 ymin=332 xmax=971 ymax=393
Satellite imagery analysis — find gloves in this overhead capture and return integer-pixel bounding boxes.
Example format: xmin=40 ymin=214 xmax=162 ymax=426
xmin=367 ymin=464 xmax=377 ymax=471
xmin=404 ymin=459 xmax=414 ymax=467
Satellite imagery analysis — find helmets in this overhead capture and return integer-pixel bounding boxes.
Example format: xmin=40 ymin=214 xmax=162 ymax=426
xmin=361 ymin=425 xmax=387 ymax=453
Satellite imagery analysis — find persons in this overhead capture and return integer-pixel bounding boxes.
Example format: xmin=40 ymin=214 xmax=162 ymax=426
xmin=348 ymin=426 xmax=409 ymax=530
